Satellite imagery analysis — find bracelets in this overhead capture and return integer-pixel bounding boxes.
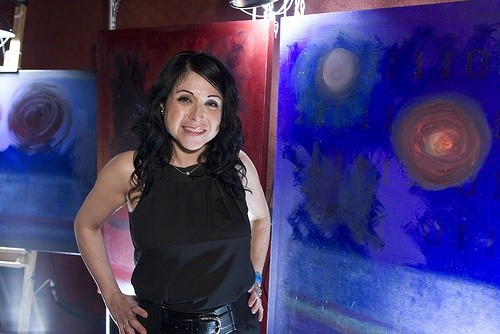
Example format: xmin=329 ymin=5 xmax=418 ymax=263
xmin=254 ymin=282 xmax=262 ymax=298
xmin=255 ymin=271 xmax=262 ymax=284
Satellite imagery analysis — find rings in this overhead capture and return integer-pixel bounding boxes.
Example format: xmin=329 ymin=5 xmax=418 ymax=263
xmin=123 ymin=322 xmax=130 ymax=330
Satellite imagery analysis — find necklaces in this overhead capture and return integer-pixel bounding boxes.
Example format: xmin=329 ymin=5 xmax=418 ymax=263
xmin=170 ymin=159 xmax=201 ymax=175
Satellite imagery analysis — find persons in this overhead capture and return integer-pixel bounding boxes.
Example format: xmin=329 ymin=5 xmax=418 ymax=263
xmin=74 ymin=52 xmax=270 ymax=334
